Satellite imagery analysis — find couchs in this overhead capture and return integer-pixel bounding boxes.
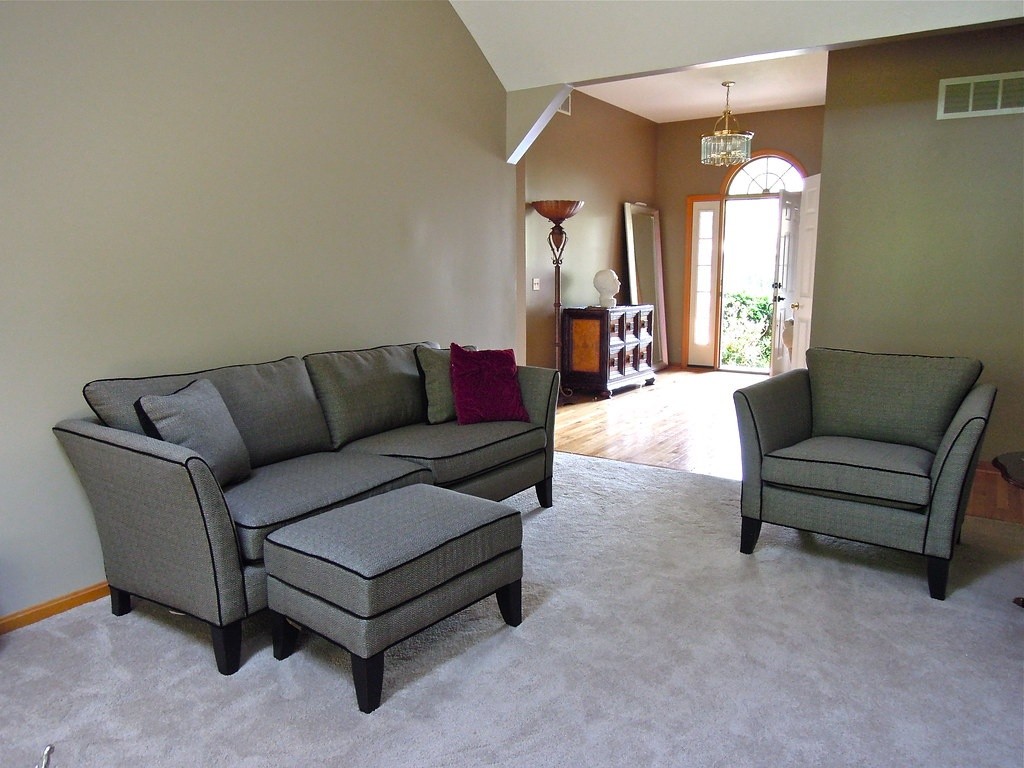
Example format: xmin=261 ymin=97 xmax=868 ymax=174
xmin=733 ymin=346 xmax=997 ymax=601
xmin=52 ymin=341 xmax=561 ymax=675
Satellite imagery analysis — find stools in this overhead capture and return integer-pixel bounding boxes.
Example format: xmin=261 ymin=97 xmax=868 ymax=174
xmin=263 ymin=482 xmax=523 ymax=714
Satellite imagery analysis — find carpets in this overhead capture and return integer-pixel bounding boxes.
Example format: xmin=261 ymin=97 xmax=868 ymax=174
xmin=0 ymin=449 xmax=1024 ymax=768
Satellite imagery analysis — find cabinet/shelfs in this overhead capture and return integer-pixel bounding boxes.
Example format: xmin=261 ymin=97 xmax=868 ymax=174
xmin=562 ymin=304 xmax=656 ymax=399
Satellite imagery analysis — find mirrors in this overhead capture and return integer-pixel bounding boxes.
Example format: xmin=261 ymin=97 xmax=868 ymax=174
xmin=625 ymin=203 xmax=668 ymax=373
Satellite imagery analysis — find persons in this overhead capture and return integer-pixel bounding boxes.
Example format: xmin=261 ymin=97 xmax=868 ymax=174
xmin=593 ymin=269 xmax=621 ymax=308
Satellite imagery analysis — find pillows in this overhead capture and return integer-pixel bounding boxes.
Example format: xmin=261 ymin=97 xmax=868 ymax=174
xmin=449 ymin=342 xmax=531 ymax=426
xmin=413 ymin=345 xmax=481 ymax=424
xmin=133 ymin=378 xmax=251 ymax=488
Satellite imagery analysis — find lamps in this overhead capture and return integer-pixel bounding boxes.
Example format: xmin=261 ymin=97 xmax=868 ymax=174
xmin=531 ymin=200 xmax=585 ymax=397
xmin=701 ymin=81 xmax=755 ymax=167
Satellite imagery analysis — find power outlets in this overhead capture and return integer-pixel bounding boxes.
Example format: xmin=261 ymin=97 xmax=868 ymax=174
xmin=532 ymin=279 xmax=541 ymax=291
